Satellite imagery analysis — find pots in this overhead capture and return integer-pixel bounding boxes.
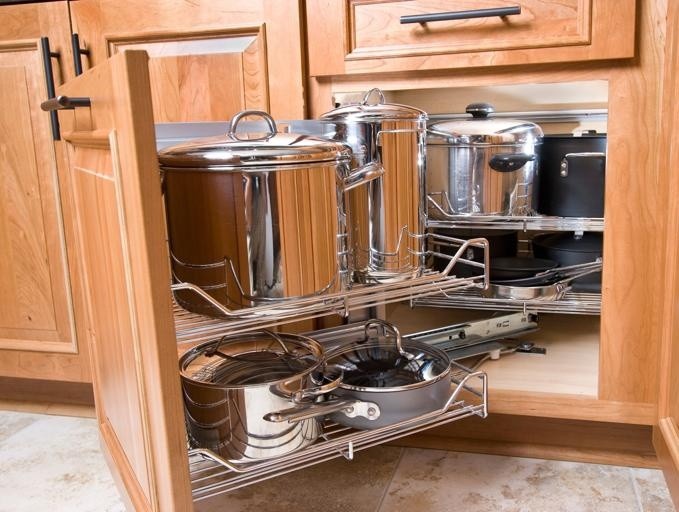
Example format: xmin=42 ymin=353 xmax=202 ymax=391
xmin=157 ymin=109 xmax=353 ymax=319
xmin=263 ymin=317 xmax=452 ymax=432
xmin=426 ymin=101 xmax=543 ymax=223
xmin=536 ymin=128 xmax=607 ymax=218
xmin=178 ymin=329 xmax=345 ymax=464
xmin=433 ymin=227 xmax=603 ymax=300
xmin=320 ymin=86 xmax=429 ymax=285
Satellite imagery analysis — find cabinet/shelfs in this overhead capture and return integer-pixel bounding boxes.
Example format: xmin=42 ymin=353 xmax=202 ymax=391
xmin=0 ymin=0 xmax=679 ymax=512
